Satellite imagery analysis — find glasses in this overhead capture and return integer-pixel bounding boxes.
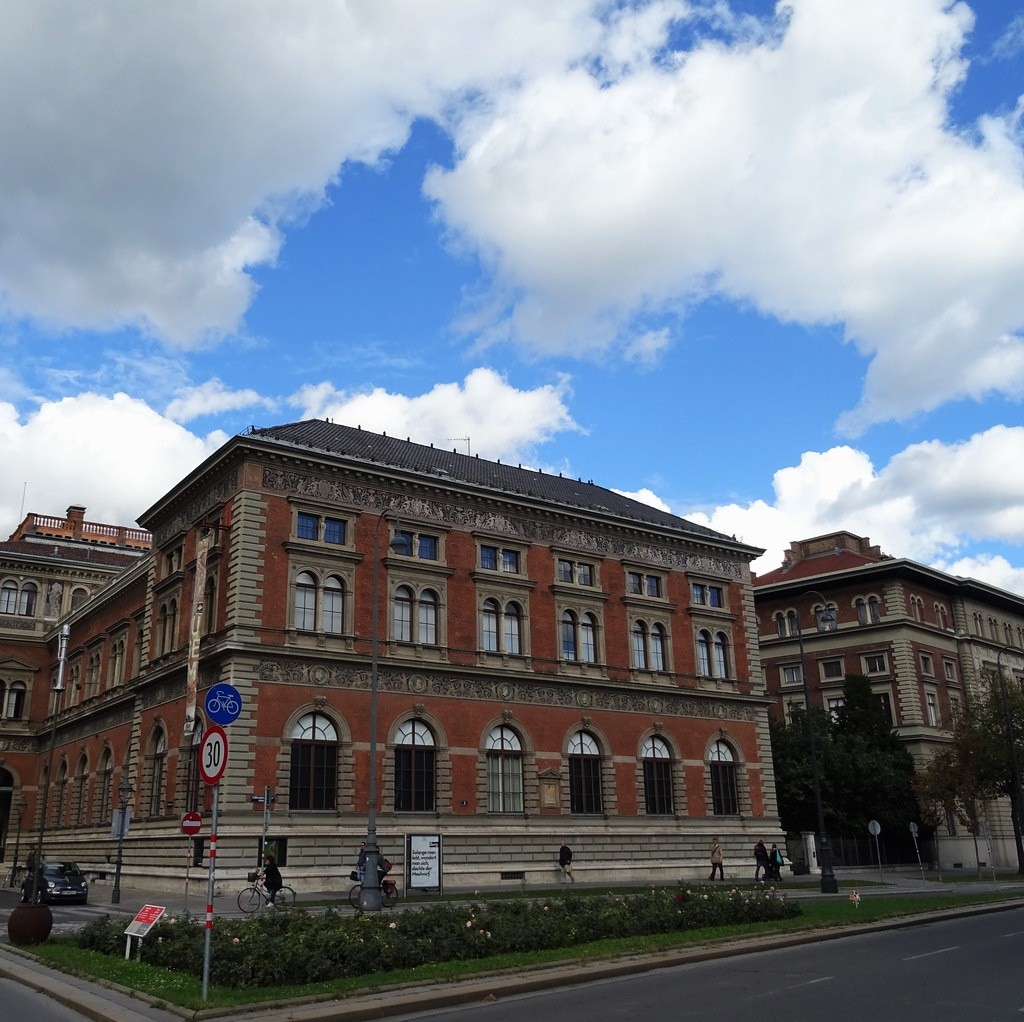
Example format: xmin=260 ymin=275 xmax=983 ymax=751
xmin=360 ymin=845 xmax=364 ymax=846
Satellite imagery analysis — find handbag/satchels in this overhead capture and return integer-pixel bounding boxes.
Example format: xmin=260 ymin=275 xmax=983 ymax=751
xmin=776 ymin=850 xmax=782 ymax=865
xmin=350 ymin=871 xmax=360 ymax=881
xmin=565 ymin=864 xmax=573 ymax=873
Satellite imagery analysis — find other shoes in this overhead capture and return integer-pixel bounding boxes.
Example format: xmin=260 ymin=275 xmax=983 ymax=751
xmin=266 ymin=902 xmax=274 ymax=907
xmin=709 ymin=877 xmax=714 ymax=881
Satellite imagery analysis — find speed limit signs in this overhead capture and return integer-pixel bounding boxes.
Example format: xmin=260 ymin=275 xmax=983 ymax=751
xmin=199 ymin=725 xmax=229 ymax=785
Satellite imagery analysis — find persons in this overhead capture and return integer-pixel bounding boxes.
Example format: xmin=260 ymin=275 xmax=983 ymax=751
xmin=258 ymin=856 xmax=282 ymax=908
xmin=753 ymin=838 xmax=783 ymax=882
xmin=708 ymin=838 xmax=724 ymax=882
xmin=26 ymin=847 xmax=46 ymax=869
xmin=355 ymin=842 xmax=388 ymax=885
xmin=559 ymin=841 xmax=575 ymax=884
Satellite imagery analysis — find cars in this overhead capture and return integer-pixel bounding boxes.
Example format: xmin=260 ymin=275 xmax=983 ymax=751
xmin=21 ymin=860 xmax=89 ymax=906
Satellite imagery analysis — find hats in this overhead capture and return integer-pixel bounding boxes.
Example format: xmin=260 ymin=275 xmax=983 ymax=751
xmin=772 ymin=844 xmax=776 ymax=848
xmin=759 ymin=840 xmax=764 ymax=844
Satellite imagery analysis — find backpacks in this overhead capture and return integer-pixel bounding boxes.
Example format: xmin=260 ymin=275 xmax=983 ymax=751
xmin=753 ymin=845 xmax=762 ymax=857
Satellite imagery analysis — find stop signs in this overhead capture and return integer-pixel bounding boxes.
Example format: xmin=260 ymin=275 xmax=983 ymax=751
xmin=181 ymin=812 xmax=202 ymax=835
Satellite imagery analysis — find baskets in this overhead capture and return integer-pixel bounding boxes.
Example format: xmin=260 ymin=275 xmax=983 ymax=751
xmin=248 ymin=872 xmax=257 ymax=882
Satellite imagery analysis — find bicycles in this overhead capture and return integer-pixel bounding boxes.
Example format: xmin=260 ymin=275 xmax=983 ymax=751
xmin=237 ymin=872 xmax=297 ymax=913
xmin=348 ymin=875 xmax=399 ymax=910
xmin=2 ymin=866 xmax=24 ymax=890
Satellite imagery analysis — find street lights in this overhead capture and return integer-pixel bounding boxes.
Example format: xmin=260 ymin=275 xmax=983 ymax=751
xmin=110 ymin=779 xmax=131 ymax=903
xmin=795 ymin=589 xmax=838 ymax=894
xmin=354 ymin=503 xmax=405 ymax=907
